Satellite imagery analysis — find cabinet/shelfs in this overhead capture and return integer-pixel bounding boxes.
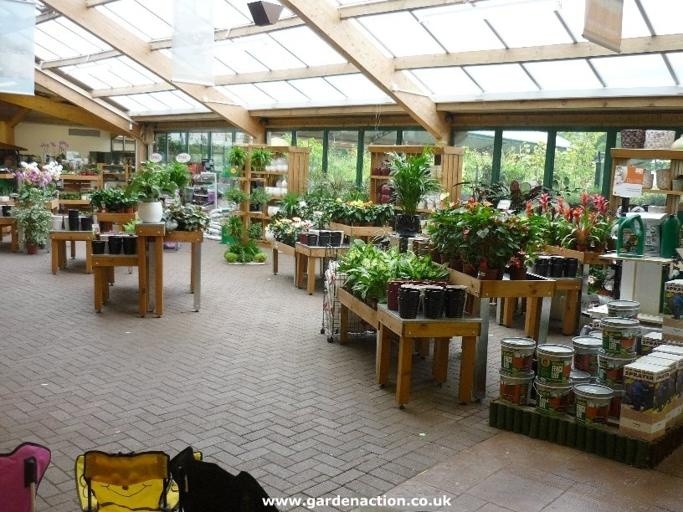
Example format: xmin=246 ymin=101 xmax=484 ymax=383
xmin=230 ymin=145 xmax=308 ymax=247
xmin=0 ymin=163 xmax=131 ymax=193
xmin=367 ymin=144 xmax=465 ymax=216
xmin=225 ymin=147 xmax=268 ymax=265
xmin=193 ymin=172 xmax=218 ymax=211
xmin=608 ymin=148 xmax=682 ymax=224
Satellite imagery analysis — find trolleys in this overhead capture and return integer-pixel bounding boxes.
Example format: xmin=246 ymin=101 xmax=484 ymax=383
xmin=320 ymin=244 xmax=369 ymax=341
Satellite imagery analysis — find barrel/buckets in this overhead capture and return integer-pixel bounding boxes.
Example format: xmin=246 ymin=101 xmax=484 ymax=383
xmin=569 ymin=368 xmax=592 ymax=384
xmin=500 ymin=337 xmax=536 ymax=373
xmin=589 ymin=330 xmax=604 ymax=338
xmin=600 ymin=317 xmax=643 ymax=358
xmin=566 ymin=368 xmax=590 ymax=415
xmin=532 ymin=375 xmax=574 ymax=413
xmin=595 ymin=378 xmax=626 ymax=420
xmin=607 ymin=299 xmax=641 ymax=319
xmin=498 ymin=369 xmax=535 ymax=405
xmin=660 ymin=213 xmax=681 ymax=258
xmin=537 ymin=344 xmax=576 ymax=384
xmin=572 ymin=335 xmax=604 ymax=377
xmin=571 ymin=383 xmax=614 ymax=432
xmin=597 ymin=348 xmax=637 ymax=386
xmin=616 ymin=213 xmax=646 ymax=257
